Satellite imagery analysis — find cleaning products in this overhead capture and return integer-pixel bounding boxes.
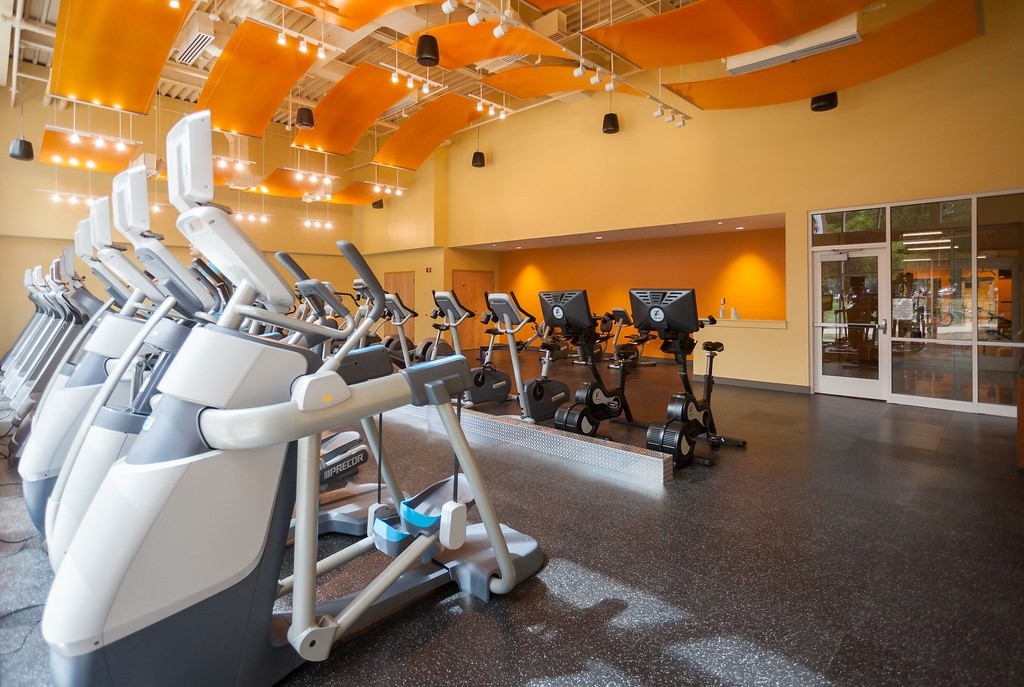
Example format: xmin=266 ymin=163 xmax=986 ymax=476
xmin=720 ymin=298 xmax=726 ymax=319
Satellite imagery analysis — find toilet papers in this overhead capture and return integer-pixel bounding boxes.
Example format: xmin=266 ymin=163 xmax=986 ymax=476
xmin=731 ymin=308 xmax=737 ymax=319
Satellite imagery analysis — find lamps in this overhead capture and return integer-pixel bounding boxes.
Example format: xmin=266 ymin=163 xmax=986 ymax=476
xmin=294 ymin=76 xmax=315 ymax=130
xmin=415 ymin=3 xmax=439 ymax=67
xmin=153 ymin=205 xmax=160 ymax=212
xmin=589 ymin=67 xmax=600 ymax=86
xmin=676 ymin=115 xmax=685 ymax=128
xmin=218 ymin=158 xmax=269 ymax=223
xmin=8 ymin=60 xmax=34 ymax=162
xmin=573 ymin=59 xmax=586 ymax=78
xmin=605 ymin=75 xmax=616 ymax=91
xmin=391 ymin=69 xmax=430 ymax=93
xmin=664 ymin=110 xmax=675 ymax=123
xmin=476 ymin=100 xmax=505 ymax=119
xmin=441 ymin=0 xmax=508 ymax=38
xmin=472 ymin=110 xmax=485 ymax=168
xmin=374 ymin=185 xmax=403 ymax=196
xmin=51 ymin=103 xmax=127 ymax=206
xmin=602 ymin=76 xmax=619 ymax=134
xmin=811 ymin=90 xmax=839 ymax=112
xmin=278 ymin=31 xmax=325 ymax=60
xmin=295 ymin=173 xmax=333 ymax=229
xmin=653 ymin=104 xmax=664 ymax=118
